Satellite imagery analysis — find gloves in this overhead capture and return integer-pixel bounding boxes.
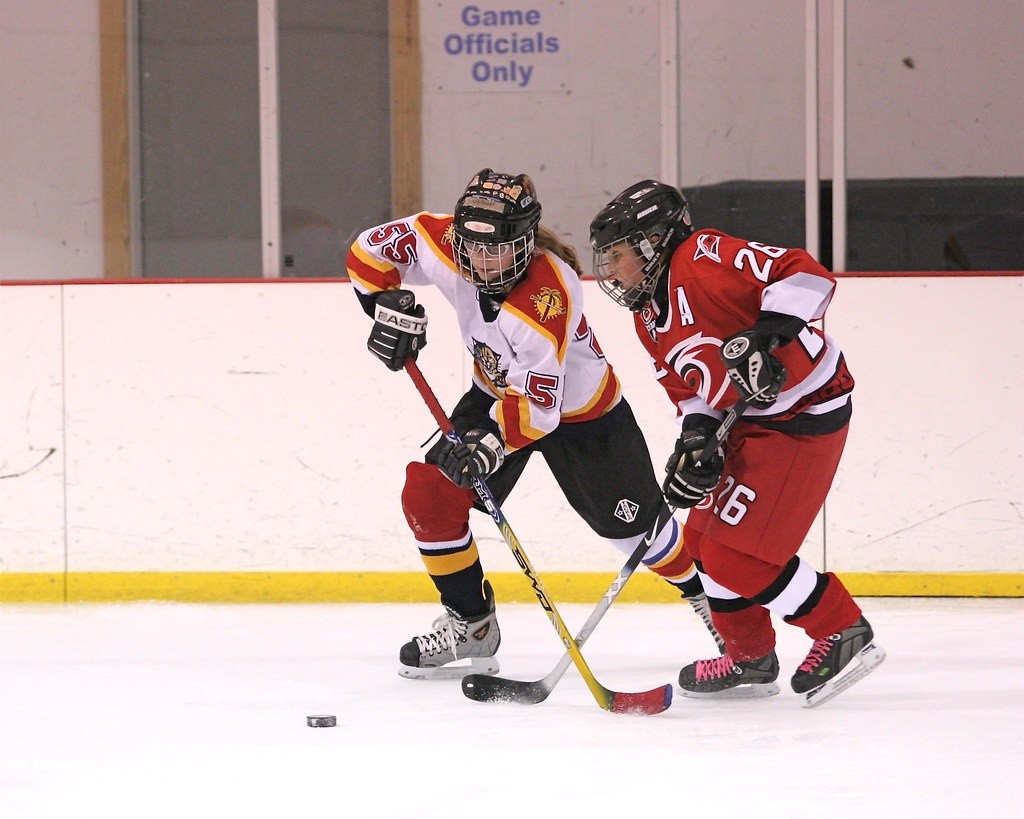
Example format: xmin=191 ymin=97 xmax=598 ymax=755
xmin=437 ymin=428 xmax=506 ymax=489
xmin=367 ymin=290 xmax=428 ymax=372
xmin=662 ymin=414 xmax=728 ymax=510
xmin=718 ymin=328 xmax=787 ymax=410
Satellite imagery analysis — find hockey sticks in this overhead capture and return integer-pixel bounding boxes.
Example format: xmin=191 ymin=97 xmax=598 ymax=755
xmin=461 ymin=337 xmax=776 ymax=705
xmin=404 ymin=356 xmax=674 ymax=715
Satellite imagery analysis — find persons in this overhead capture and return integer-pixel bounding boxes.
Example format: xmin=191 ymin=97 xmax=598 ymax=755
xmin=347 ymin=168 xmax=725 ymax=668
xmin=591 ymin=180 xmax=875 ymax=694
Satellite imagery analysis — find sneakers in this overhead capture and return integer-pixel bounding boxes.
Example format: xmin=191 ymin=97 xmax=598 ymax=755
xmin=681 ymin=591 xmax=727 ymax=656
xmin=397 ymin=580 xmax=501 ymax=680
xmin=676 ymin=648 xmax=779 ymax=699
xmin=784 ymin=572 xmax=886 ymax=709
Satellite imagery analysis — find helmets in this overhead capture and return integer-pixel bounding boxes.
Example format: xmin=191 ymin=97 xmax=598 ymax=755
xmin=450 ymin=168 xmax=541 ymax=294
xmin=590 ymin=180 xmax=694 ymax=313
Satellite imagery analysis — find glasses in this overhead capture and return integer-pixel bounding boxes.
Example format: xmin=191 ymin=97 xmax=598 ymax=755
xmin=463 ymin=240 xmax=512 ymax=257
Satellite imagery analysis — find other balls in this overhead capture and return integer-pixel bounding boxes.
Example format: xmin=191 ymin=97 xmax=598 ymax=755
xmin=307 ymin=713 xmax=337 ymax=729
